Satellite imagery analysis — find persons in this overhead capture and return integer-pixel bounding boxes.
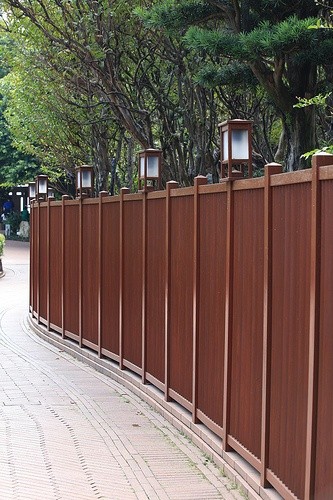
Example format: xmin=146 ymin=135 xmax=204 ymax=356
xmin=0 ymin=195 xmax=15 ymax=279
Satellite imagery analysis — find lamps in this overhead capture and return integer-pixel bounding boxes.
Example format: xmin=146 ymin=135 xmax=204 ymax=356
xmin=217 ymin=118 xmax=253 ymax=181
xmin=74 ymin=164 xmax=95 ymax=199
xmin=136 ymin=149 xmax=162 ymax=192
xmin=27 ymin=181 xmax=36 ymax=200
xmin=46 ymin=188 xmax=55 ymax=200
xmin=34 ymin=174 xmax=48 ymax=203
